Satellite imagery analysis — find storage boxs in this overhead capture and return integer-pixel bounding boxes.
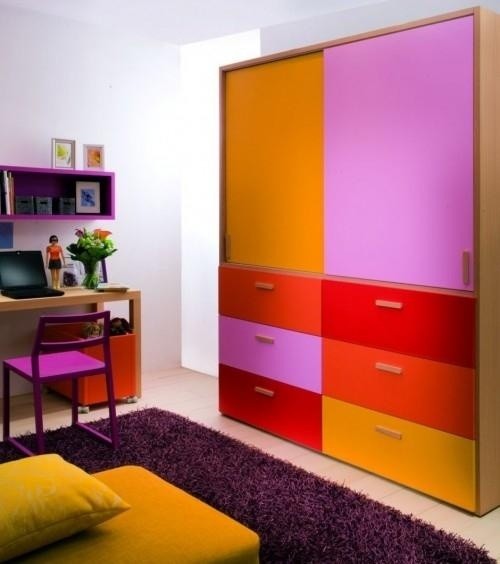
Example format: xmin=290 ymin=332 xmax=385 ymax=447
xmin=14 ymin=195 xmax=76 ymax=215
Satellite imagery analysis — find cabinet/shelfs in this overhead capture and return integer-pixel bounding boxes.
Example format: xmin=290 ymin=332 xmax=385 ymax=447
xmin=0 ymin=164 xmax=115 ymax=222
xmin=219 ymin=5 xmax=500 ymax=516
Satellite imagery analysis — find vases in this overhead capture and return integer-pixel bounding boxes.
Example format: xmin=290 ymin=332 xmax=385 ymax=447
xmin=83 ymin=261 xmax=99 ymax=288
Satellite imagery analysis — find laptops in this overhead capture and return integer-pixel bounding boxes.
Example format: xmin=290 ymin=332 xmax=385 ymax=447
xmin=1 ymin=249 xmax=66 ymax=300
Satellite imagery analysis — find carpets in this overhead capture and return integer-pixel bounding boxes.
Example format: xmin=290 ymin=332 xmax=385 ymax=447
xmin=0 ymin=406 xmax=493 ymax=564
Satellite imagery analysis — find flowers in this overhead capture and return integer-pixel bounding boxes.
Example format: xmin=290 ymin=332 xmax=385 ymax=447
xmin=66 ymin=226 xmax=117 ymax=287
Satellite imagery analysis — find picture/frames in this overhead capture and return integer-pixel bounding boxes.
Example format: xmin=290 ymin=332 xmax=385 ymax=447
xmin=74 ymin=181 xmax=101 ymax=213
xmin=83 ymin=142 xmax=105 ymax=172
xmin=51 ymin=138 xmax=76 ymax=170
xmin=97 ymin=258 xmax=107 ymax=283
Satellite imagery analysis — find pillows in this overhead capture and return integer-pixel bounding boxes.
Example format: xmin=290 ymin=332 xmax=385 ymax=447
xmin=0 ymin=453 xmax=129 ymax=563
xmin=12 ymin=466 xmax=261 ymax=563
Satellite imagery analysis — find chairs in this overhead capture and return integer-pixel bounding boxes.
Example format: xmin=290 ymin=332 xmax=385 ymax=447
xmin=3 ymin=309 xmax=120 ymax=458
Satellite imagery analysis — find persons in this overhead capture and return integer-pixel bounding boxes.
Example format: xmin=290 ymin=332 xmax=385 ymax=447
xmin=45 ymin=234 xmax=67 ymax=288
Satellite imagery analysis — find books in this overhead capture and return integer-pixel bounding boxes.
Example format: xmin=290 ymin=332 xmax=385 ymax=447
xmin=96 ymin=281 xmax=130 ymax=289
xmin=3 ymin=170 xmax=15 ymax=214
xmin=103 ymin=288 xmax=127 ymax=292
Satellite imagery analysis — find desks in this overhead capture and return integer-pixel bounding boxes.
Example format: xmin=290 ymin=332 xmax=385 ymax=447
xmin=1 ymin=282 xmax=141 ymax=414
